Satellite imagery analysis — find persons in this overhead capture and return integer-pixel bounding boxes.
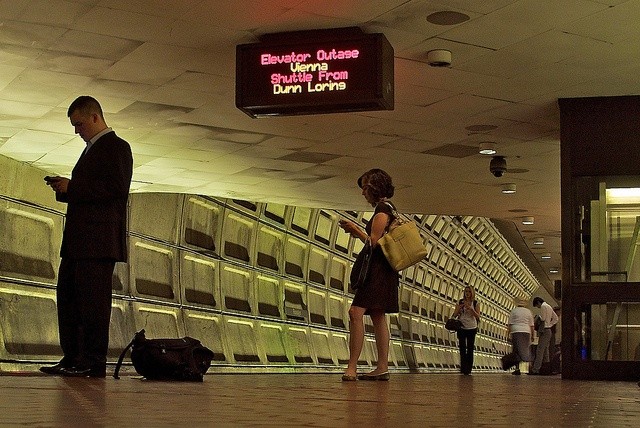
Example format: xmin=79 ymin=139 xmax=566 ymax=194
xmin=38 ymin=94 xmax=134 ymax=378
xmin=453 ymin=286 xmax=480 ymax=376
xmin=528 ymin=297 xmax=559 ymax=375
xmin=506 ymin=296 xmax=535 ymax=375
xmin=337 ymin=168 xmax=400 ymax=382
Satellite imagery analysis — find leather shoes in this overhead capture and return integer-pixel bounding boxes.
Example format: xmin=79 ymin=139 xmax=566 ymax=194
xmin=342 ymin=374 xmax=357 ymax=381
xmin=59 ymin=366 xmax=106 ymax=377
xmin=358 ymin=371 xmax=390 ymax=380
xmin=39 ymin=362 xmax=60 ymax=374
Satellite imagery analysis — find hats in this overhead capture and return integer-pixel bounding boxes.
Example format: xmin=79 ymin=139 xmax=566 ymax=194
xmin=513 ymin=296 xmax=531 ymax=308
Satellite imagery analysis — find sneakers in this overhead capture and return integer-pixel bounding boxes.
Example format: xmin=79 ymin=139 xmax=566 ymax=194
xmin=467 ymin=369 xmax=472 ymax=375
xmin=540 ymin=371 xmax=552 ymax=375
xmin=512 ymin=369 xmax=521 ymax=375
xmin=526 ymin=371 xmax=540 ymax=375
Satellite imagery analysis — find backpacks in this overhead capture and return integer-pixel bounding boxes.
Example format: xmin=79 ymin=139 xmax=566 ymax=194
xmin=112 ymin=329 xmax=215 ymax=382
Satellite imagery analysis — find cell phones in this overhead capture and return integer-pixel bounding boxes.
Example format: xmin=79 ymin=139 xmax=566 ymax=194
xmin=44 ymin=176 xmax=53 ymax=183
xmin=339 ymin=220 xmax=345 ymax=224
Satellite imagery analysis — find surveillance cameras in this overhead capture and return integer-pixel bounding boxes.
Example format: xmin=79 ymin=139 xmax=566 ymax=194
xmin=489 ymin=156 xmax=507 ymax=177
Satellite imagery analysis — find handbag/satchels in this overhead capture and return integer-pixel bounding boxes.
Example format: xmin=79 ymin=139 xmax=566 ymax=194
xmin=445 ymin=313 xmax=464 ymax=332
xmin=376 ymin=201 xmax=428 ymax=273
xmin=502 ymin=339 xmax=522 ymax=371
xmin=351 ymin=236 xmax=373 ymax=291
